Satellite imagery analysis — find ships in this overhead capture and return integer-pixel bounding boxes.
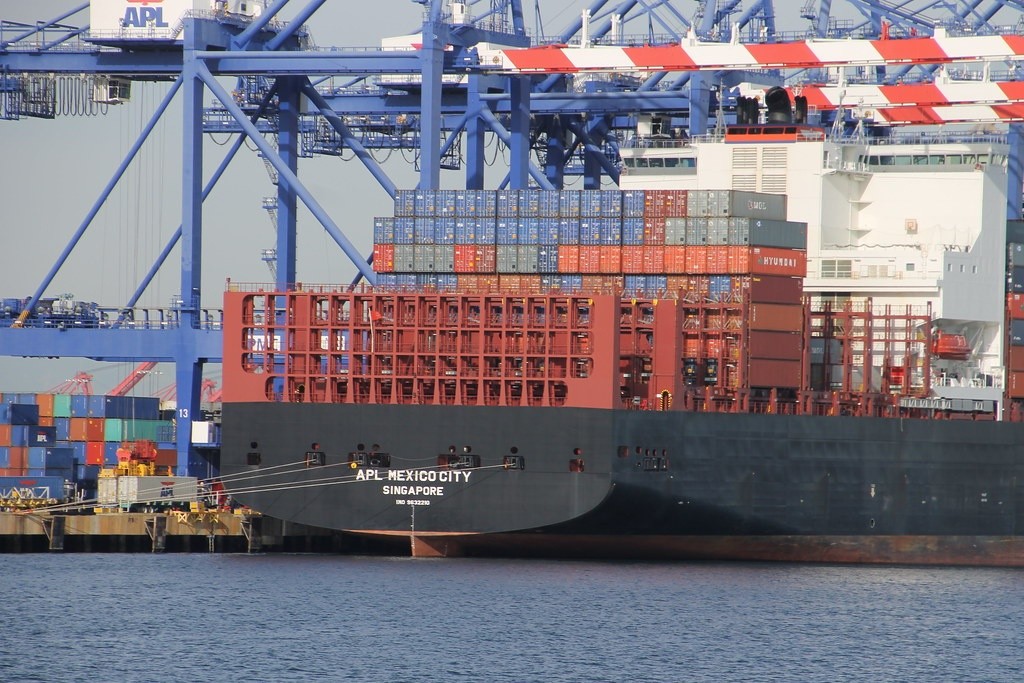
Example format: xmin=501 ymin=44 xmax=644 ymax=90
xmin=219 ymin=80 xmax=1024 ymax=568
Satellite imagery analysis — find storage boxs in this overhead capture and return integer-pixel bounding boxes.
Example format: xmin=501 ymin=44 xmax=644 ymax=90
xmin=0 ymin=393 xmax=197 ymax=504
xmin=373 ymin=189 xmax=807 ymax=387
xmin=93 ymin=507 xmax=118 ymax=515
xmin=1008 ymin=242 xmax=1024 ymax=399
xmin=190 ymin=501 xmax=249 ymax=515
